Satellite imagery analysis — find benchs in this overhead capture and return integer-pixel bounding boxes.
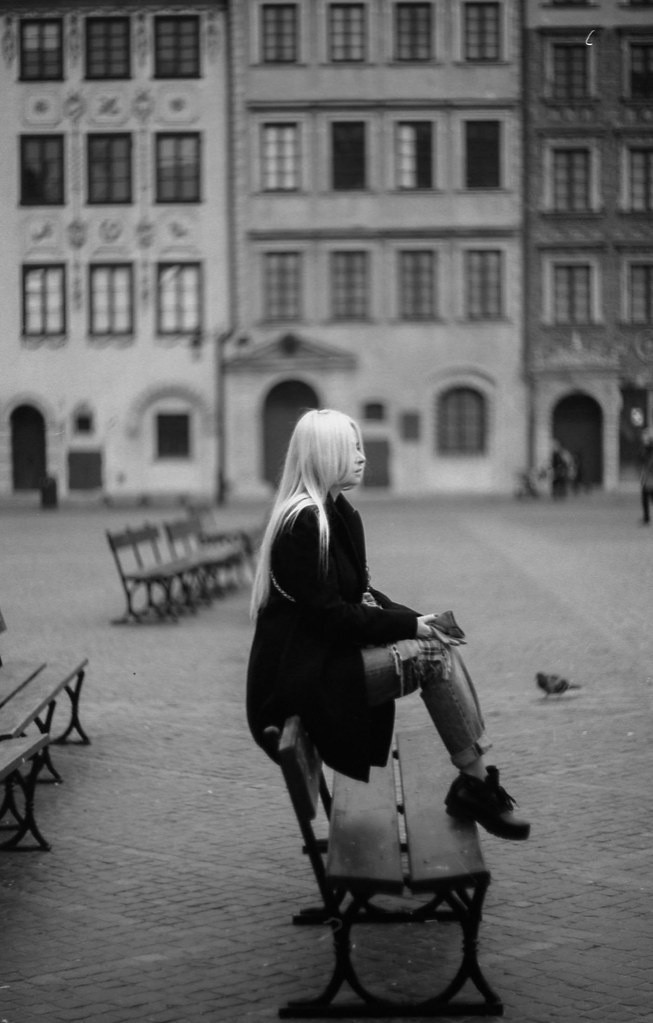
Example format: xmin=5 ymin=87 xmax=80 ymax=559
xmin=0 ymin=610 xmax=95 ymax=855
xmin=265 ymin=702 xmax=506 ymax=1019
xmin=108 ymin=500 xmax=257 ymax=627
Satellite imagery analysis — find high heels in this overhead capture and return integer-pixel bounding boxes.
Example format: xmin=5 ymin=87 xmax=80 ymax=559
xmin=445 ymin=765 xmax=531 ymax=841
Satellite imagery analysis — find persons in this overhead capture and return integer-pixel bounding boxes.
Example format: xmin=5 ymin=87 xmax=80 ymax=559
xmin=539 ymin=437 xmax=576 ymax=501
xmin=244 ymin=409 xmax=531 ymax=841
xmin=636 ymin=428 xmax=653 ymax=524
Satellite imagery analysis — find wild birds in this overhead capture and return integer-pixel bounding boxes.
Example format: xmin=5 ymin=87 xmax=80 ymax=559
xmin=535 ymin=671 xmax=584 ymax=701
